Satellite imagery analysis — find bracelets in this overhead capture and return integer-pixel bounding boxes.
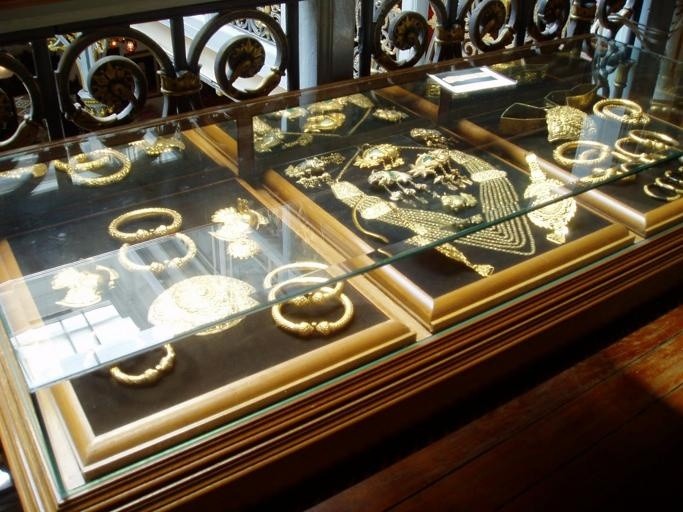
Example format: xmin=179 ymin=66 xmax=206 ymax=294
xmin=1 ymin=162 xmax=46 ymax=179
xmin=53 ymin=263 xmax=122 ymax=307
xmin=119 ymin=233 xmax=198 ymax=273
xmin=550 ymin=97 xmax=683 ymax=204
xmin=54 ymin=156 xmax=109 ymax=171
xmin=69 ymin=150 xmax=131 ymax=187
xmin=106 ymin=205 xmax=184 ymax=241
xmin=427 ymin=61 xmax=550 ymax=95
xmin=264 ymin=259 xmax=344 ymax=308
xmin=105 ymin=345 xmax=179 ymax=388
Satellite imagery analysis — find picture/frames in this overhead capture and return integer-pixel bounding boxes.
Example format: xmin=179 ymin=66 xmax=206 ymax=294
xmin=124 ymin=51 xmax=162 ymax=101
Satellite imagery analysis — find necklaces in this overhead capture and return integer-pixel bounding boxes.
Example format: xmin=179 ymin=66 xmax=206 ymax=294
xmin=332 ymin=140 xmax=537 ymax=280
xmin=250 ymin=92 xmax=374 ymax=153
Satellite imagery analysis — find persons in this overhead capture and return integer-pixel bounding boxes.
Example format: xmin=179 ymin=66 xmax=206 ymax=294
xmin=271 ymin=287 xmax=356 ymax=337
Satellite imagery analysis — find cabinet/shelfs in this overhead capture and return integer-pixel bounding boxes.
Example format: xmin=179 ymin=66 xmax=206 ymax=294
xmin=0 ymin=34 xmax=683 ymax=512
